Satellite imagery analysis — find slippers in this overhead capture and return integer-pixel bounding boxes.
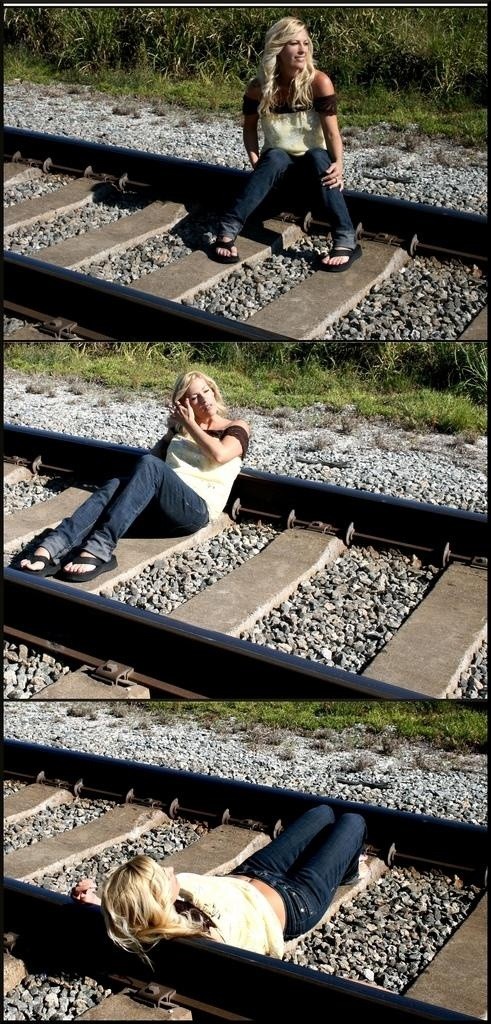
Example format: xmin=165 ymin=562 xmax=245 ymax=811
xmin=20 ymin=552 xmax=61 ymax=577
xmin=322 ymin=245 xmax=363 ymax=272
xmin=60 ymin=554 xmax=117 ymax=583
xmin=216 ymin=240 xmax=240 ymax=264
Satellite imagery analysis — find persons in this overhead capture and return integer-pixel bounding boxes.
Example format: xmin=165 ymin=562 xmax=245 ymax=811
xmin=212 ymin=17 xmax=363 ymax=272
xmin=72 ymin=805 xmax=368 ymax=971
xmin=17 ymin=370 xmax=250 ymax=583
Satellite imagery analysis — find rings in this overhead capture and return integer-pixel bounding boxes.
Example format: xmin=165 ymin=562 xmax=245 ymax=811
xmin=335 ymin=177 xmax=338 ymax=183
